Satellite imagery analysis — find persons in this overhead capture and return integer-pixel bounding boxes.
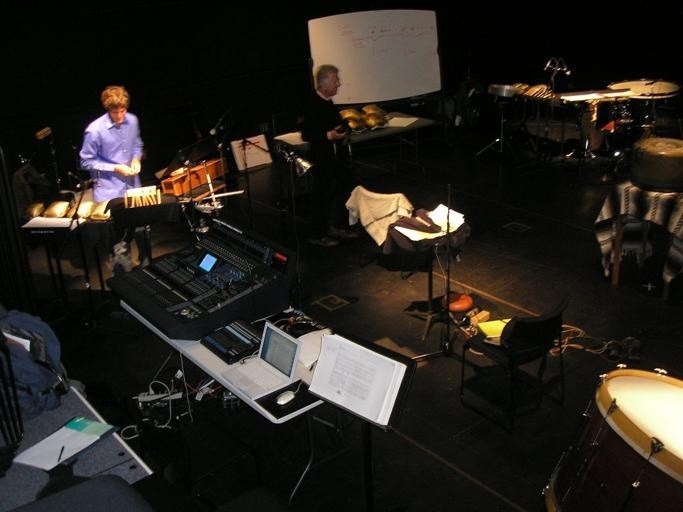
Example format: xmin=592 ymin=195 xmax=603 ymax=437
xmin=300 ymin=66 xmax=360 ymax=249
xmin=77 ymin=84 xmax=143 ymax=272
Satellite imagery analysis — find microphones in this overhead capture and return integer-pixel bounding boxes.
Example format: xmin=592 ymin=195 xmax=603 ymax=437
xmin=97 ymin=169 xmax=102 ymax=187
xmin=209 ymin=115 xmax=225 ymax=135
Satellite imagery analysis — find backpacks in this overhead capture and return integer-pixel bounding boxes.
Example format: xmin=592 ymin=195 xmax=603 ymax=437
xmin=0 ymin=309 xmax=71 ymax=423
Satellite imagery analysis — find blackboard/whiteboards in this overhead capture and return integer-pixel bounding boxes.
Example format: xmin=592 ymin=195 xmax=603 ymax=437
xmin=307 ymin=10 xmax=441 ymax=105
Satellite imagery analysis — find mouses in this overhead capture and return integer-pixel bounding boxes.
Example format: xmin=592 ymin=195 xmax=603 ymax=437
xmin=276 ymin=390 xmax=294 ymax=406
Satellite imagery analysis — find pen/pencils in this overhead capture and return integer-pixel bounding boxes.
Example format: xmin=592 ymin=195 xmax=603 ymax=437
xmin=58 ymin=446 xmax=64 ymax=461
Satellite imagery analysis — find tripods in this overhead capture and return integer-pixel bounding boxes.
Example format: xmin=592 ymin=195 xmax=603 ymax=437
xmin=475 ymin=97 xmax=517 ymax=168
xmin=404 ymin=255 xmax=446 ymax=340
xmin=403 ymin=183 xmax=486 ymax=374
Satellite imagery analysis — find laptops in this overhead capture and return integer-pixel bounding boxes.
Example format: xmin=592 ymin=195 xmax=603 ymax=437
xmin=220 ymin=320 xmax=302 ymax=400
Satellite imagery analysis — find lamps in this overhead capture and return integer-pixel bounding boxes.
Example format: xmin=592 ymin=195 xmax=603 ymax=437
xmin=275 ymin=143 xmax=314 ymax=225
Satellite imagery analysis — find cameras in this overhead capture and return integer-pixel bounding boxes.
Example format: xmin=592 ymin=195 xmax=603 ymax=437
xmin=331 ymin=120 xmax=352 ymax=135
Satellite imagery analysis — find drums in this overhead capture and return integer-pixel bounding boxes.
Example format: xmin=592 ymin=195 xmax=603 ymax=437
xmin=542 ymin=363 xmax=682 ymax=511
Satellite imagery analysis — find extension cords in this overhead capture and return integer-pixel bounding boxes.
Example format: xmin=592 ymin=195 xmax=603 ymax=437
xmin=138 ymin=390 xmax=182 ymax=403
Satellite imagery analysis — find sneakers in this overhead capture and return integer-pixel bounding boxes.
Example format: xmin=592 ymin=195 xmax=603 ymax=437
xmin=309 ymin=235 xmax=339 ymax=247
xmin=328 ymin=225 xmax=357 ymax=239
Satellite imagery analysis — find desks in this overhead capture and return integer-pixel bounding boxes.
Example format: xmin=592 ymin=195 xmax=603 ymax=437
xmin=334 ymin=112 xmax=434 ymax=183
xmin=119 ymin=298 xmax=355 ymax=511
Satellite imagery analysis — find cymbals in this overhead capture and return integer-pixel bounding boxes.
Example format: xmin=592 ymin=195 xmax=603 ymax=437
xmin=608 ymin=80 xmax=679 ymax=101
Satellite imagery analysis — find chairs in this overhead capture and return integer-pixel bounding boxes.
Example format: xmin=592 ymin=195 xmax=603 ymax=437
xmin=179 ymin=179 xmax=225 ymax=242
xmin=448 ymin=291 xmax=571 ymax=431
xmin=608 ymin=182 xmax=683 ymax=308
